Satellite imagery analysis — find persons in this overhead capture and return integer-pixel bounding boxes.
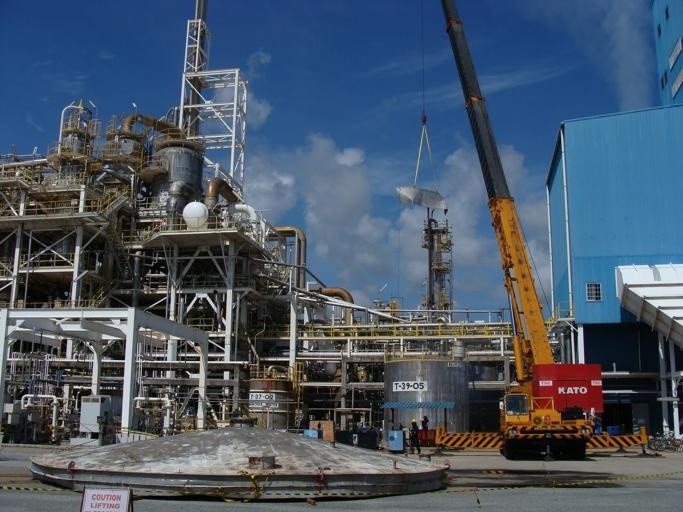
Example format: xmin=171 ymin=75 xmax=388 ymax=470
xmin=408 ymin=419 xmax=421 ymax=454
xmin=422 ymin=416 xmax=429 ymax=431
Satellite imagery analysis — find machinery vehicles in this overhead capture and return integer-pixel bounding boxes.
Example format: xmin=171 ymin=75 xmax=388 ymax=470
xmin=397 ymin=1 xmax=652 ymax=462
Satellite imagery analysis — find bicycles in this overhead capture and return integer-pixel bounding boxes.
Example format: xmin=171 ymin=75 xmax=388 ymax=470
xmin=648 ymin=427 xmax=680 ymax=455
